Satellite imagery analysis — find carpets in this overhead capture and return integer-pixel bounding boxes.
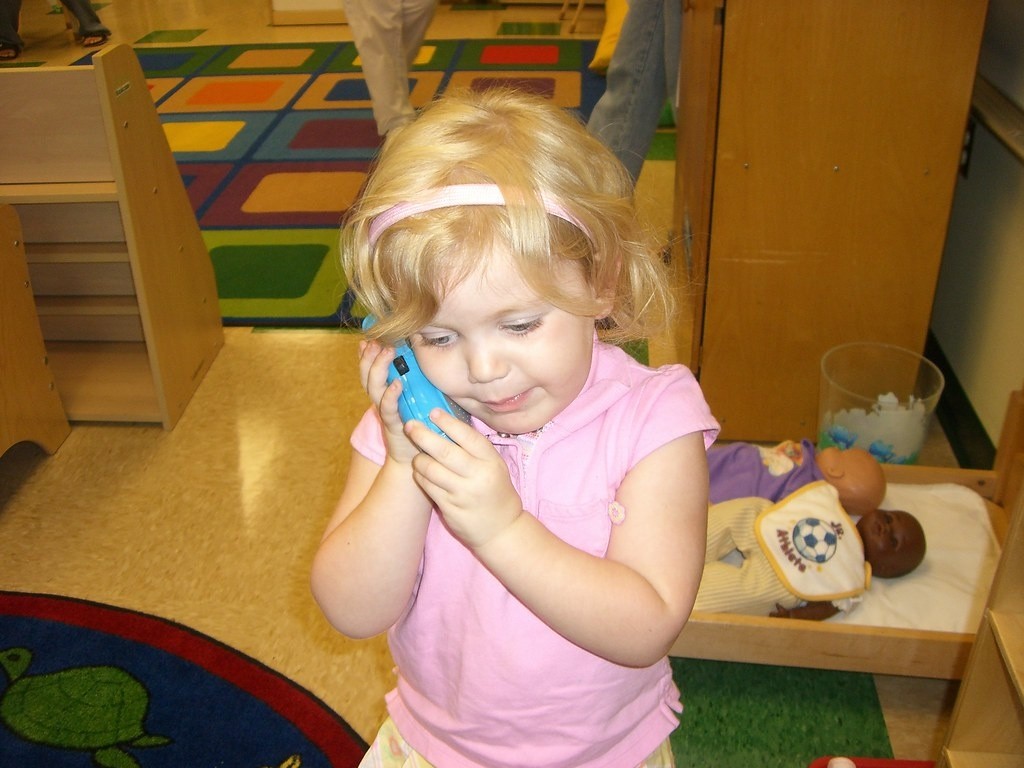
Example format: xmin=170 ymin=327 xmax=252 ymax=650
xmin=0 ymin=588 xmax=369 ymax=768
xmin=67 ymin=37 xmax=679 ymax=331
xmin=662 ymin=656 xmax=893 ymax=768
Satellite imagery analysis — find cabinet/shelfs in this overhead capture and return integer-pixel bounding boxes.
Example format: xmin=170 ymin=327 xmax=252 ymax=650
xmin=0 ymin=45 xmax=224 ymax=462
xmin=673 ymin=0 xmax=990 ymax=443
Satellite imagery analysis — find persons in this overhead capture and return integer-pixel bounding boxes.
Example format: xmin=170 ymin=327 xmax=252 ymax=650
xmin=691 ymin=478 xmax=926 ymax=622
xmin=0 ymin=0 xmax=112 ymax=61
xmin=309 ymin=85 xmax=725 ymax=768
xmin=585 ymin=1 xmax=684 ymax=195
xmin=345 ymin=0 xmax=439 ymax=135
xmin=705 ymin=436 xmax=886 ymax=515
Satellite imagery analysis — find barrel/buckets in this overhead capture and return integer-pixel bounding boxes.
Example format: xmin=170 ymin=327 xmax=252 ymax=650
xmin=818 ymin=339 xmax=945 ymax=464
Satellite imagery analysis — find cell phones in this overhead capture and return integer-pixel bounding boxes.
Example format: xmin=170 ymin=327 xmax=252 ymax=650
xmin=362 ymin=314 xmax=474 ymax=448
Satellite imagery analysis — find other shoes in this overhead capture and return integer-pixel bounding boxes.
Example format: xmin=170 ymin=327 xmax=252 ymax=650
xmin=0 ymin=46 xmax=17 ymax=59
xmin=82 ymin=34 xmax=108 ymax=47
